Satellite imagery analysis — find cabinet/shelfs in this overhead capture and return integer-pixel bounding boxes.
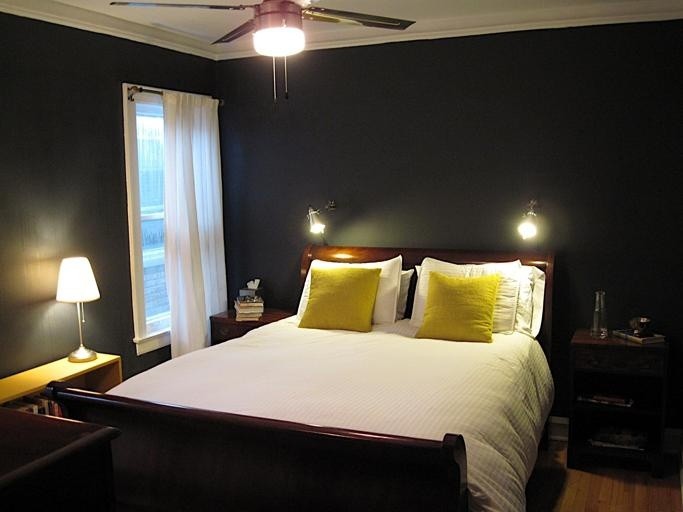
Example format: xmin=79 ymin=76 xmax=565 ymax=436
xmin=0 ymin=352 xmax=122 ymax=408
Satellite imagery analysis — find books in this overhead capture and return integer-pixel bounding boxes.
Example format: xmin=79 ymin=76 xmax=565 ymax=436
xmin=233 ymin=295 xmax=264 ymax=322
xmin=578 ymin=390 xmax=645 ymax=451
xmin=547 ymin=416 xmax=569 ymax=442
xmin=5 ymin=394 xmax=62 ymax=417
xmin=612 ymin=328 xmax=666 ymax=344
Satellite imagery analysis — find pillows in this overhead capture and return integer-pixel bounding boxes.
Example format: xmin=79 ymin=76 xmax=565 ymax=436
xmin=299 ymin=254 xmax=544 ymax=342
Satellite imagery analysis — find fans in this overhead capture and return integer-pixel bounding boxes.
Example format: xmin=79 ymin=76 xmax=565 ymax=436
xmin=109 ymin=0 xmax=415 ymax=45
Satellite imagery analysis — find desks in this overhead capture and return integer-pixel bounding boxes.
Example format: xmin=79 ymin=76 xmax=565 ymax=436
xmin=1 ymin=407 xmax=121 ymax=512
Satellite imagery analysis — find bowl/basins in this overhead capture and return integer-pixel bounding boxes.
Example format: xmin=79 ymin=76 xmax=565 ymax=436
xmin=628 ymin=317 xmax=651 ymax=337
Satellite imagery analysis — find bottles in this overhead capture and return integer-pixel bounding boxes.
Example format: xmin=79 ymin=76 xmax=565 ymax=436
xmin=590 ymin=291 xmax=608 ymax=340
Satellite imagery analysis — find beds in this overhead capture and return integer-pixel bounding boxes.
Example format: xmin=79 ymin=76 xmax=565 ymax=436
xmin=43 ymin=244 xmax=567 ymax=512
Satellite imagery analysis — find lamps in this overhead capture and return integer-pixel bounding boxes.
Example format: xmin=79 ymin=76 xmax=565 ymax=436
xmin=520 ymin=196 xmax=543 ymax=241
xmin=54 ymin=257 xmax=101 ymax=363
xmin=254 ymin=1 xmax=302 ymax=103
xmin=307 ymin=200 xmax=336 ymax=235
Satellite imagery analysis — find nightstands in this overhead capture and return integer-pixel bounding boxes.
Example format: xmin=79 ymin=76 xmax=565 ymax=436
xmin=568 ymin=328 xmax=670 ymax=471
xmin=210 ymin=308 xmax=297 ymax=346
